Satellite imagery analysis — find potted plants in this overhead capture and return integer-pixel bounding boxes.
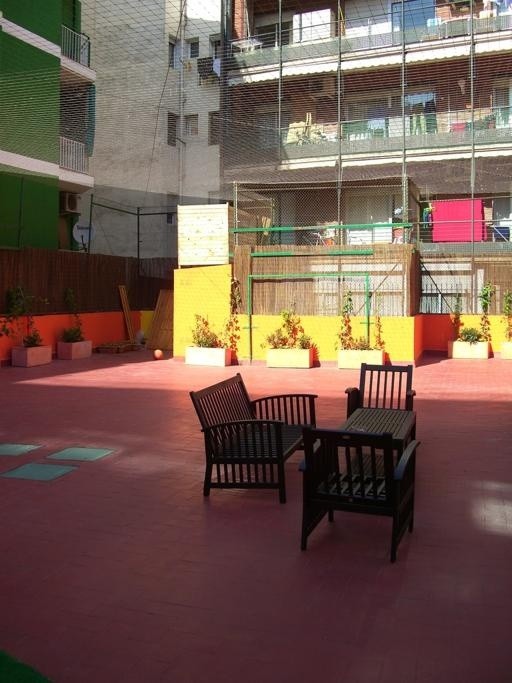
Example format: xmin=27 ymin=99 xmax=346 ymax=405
xmin=447 ymin=283 xmax=512 ymax=359
xmin=185 ymin=291 xmax=386 ymax=369
xmin=94 ymin=340 xmax=142 ymax=354
xmin=1 ymin=286 xmax=92 ymax=367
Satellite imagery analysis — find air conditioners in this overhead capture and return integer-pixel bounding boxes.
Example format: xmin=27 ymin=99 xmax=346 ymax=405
xmin=60 ymin=192 xmax=80 ymax=217
xmin=308 ymin=77 xmax=335 ymax=97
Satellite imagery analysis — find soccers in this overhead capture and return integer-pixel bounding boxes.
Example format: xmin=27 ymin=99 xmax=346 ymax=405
xmin=154 ymin=350 xmax=163 ymax=358
